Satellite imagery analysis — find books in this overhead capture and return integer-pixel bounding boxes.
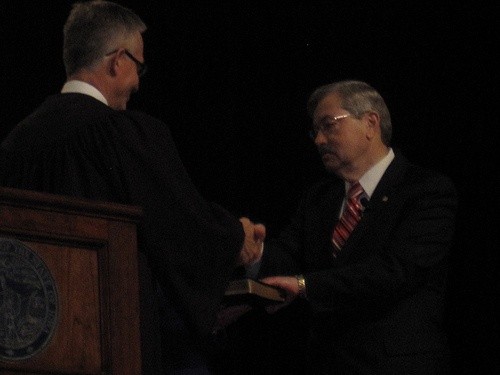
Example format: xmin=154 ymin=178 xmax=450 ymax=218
xmin=209 ymin=278 xmax=287 ymax=310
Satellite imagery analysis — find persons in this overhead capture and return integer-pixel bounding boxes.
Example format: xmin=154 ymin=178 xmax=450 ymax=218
xmin=0 ymin=0 xmax=259 ymax=375
xmin=239 ymin=81 xmax=458 ymax=375
xmin=170 ymin=303 xmax=253 ymax=375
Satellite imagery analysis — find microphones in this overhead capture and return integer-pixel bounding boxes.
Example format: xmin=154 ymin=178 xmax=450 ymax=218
xmin=358 ymin=196 xmax=374 ymax=222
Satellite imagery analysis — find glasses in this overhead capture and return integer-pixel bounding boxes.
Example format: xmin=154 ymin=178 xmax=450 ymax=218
xmin=104 ymin=46 xmax=148 ymax=74
xmin=309 ymin=111 xmax=350 ymax=138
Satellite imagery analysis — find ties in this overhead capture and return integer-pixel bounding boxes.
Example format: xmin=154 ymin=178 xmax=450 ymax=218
xmin=330 ymin=183 xmax=365 ymax=258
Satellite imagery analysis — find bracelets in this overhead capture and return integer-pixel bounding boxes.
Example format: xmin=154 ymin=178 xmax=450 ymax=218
xmin=296 ymin=274 xmax=305 ymax=299
